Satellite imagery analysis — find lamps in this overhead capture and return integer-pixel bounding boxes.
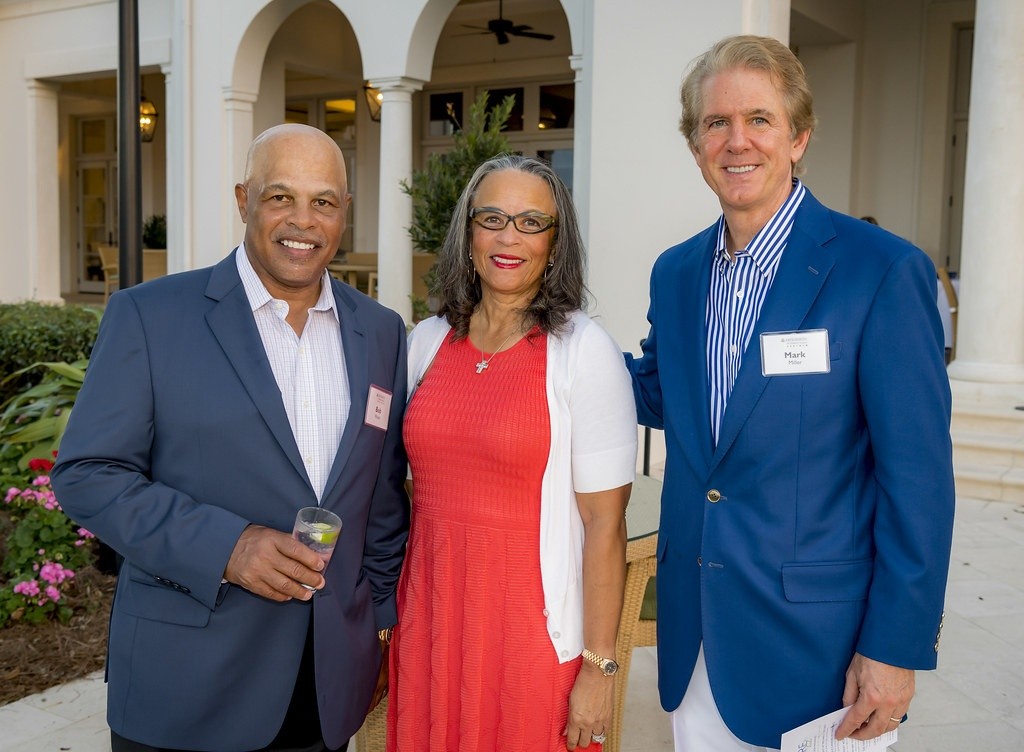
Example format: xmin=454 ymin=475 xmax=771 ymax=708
xmin=139 ymin=90 xmax=159 ymax=144
xmin=361 ymin=78 xmax=382 ymax=124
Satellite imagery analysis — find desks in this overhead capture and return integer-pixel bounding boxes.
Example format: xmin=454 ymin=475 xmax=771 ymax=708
xmin=326 ymin=264 xmax=378 ymax=290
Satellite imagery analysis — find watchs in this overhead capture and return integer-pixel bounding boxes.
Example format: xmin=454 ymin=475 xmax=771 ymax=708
xmin=376 ymin=629 xmax=393 ymax=644
xmin=581 ymin=646 xmax=621 ymax=676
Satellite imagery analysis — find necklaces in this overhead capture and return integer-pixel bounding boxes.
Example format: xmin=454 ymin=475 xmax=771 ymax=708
xmin=474 ymin=301 xmax=532 ymax=374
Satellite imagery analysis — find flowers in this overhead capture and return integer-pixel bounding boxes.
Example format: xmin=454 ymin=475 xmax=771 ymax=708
xmin=0 ymin=450 xmax=97 ymax=627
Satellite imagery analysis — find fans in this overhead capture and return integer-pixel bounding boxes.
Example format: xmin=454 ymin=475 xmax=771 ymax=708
xmin=457 ymin=0 xmax=557 ymax=47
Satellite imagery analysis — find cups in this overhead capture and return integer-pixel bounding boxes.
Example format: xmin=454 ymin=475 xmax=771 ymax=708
xmin=287 ymin=504 xmax=344 ymax=596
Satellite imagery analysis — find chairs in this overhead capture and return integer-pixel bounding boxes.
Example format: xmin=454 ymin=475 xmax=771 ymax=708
xmin=97 ymin=245 xmax=120 ymax=307
xmin=140 ymin=248 xmax=167 ymax=283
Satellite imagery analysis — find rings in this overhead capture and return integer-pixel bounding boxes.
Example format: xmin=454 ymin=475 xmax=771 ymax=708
xmin=592 ymin=731 xmax=606 ymax=744
xmin=890 ymin=717 xmax=903 ymax=723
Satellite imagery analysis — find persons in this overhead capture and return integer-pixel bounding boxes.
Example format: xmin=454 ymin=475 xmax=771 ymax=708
xmin=615 ymin=36 xmax=956 ymax=750
xmin=49 ymin=124 xmax=413 ymax=752
xmin=403 ymin=153 xmax=639 ymax=752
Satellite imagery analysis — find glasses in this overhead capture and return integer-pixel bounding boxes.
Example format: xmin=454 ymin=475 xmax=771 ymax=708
xmin=467 ymin=207 xmax=559 ymax=235
